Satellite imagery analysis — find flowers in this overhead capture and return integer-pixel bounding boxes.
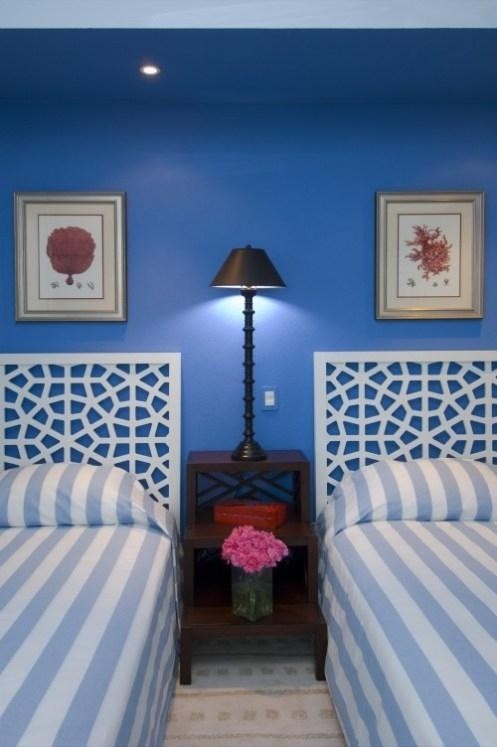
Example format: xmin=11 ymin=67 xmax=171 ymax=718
xmin=220 ymin=523 xmax=290 ymax=620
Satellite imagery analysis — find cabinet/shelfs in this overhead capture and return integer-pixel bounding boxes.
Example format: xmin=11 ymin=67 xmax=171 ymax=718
xmin=180 ymin=451 xmax=327 ymax=684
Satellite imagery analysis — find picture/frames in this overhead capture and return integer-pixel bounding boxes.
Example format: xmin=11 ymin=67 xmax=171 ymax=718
xmin=375 ymin=192 xmax=485 ymax=320
xmin=13 ymin=192 xmax=125 ymax=323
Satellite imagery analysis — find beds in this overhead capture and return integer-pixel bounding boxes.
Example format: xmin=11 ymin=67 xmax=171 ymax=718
xmin=0 ymin=354 xmax=181 ymax=745
xmin=315 ymin=351 xmax=496 ymax=747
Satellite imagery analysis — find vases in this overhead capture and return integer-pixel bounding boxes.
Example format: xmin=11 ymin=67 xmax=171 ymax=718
xmin=231 ymin=564 xmax=274 ymax=620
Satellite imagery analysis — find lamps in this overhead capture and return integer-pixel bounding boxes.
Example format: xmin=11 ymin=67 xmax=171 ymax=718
xmin=209 ymin=245 xmax=288 ymax=460
